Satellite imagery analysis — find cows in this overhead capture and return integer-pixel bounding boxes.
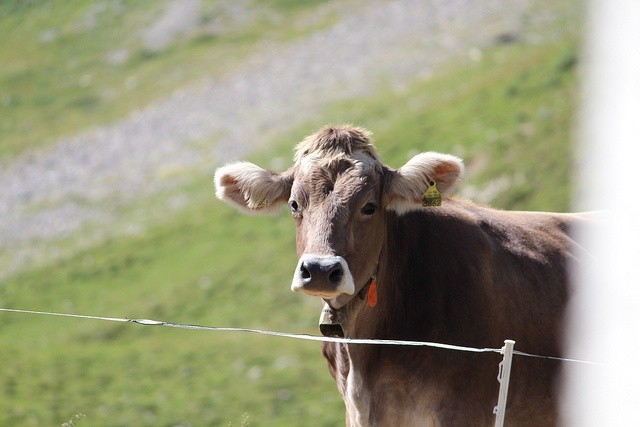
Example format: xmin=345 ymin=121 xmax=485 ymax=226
xmin=213 ymin=124 xmax=608 ymax=427
